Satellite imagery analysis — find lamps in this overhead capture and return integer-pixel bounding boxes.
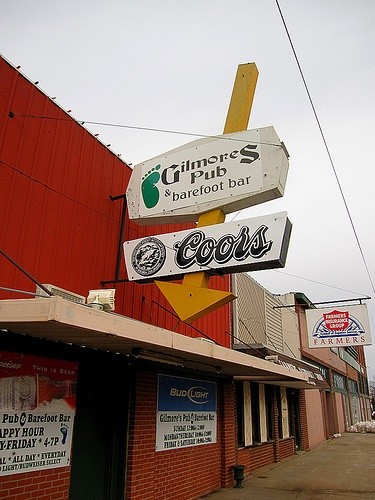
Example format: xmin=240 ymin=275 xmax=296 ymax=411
xmin=87 ymin=289 xmax=116 ymax=312
xmin=131 ymin=348 xmax=184 ymax=368
xmin=265 ymin=355 xmax=281 ymax=364
